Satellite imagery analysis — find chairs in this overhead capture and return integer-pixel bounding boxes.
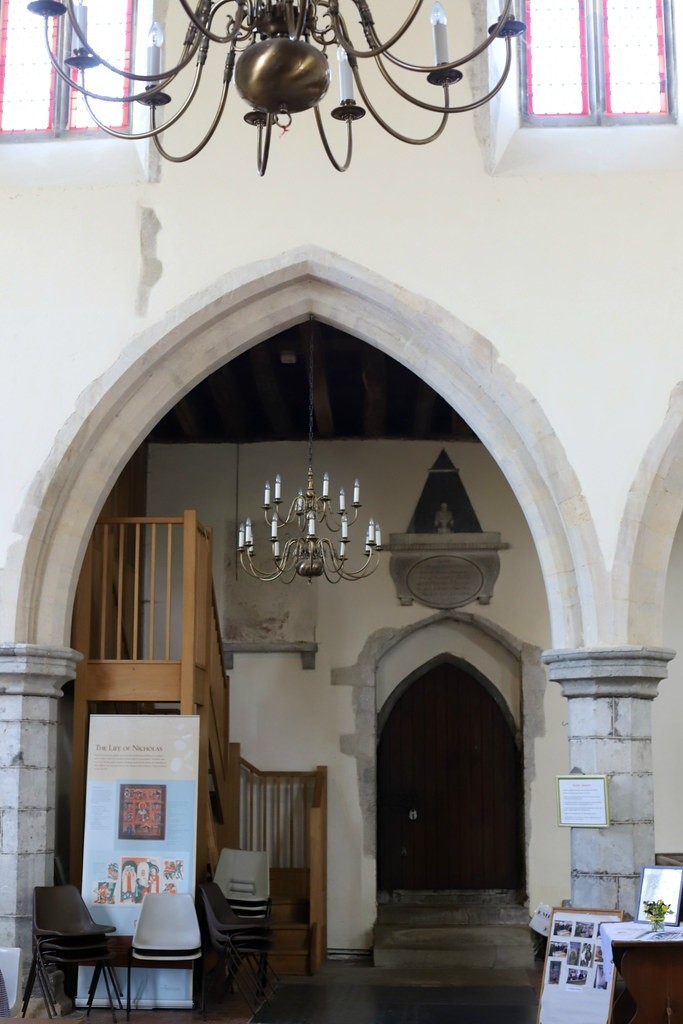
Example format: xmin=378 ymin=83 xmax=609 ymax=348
xmin=0 ymin=946 xmax=23 ymax=1017
xmin=20 ymin=847 xmax=281 ymax=1024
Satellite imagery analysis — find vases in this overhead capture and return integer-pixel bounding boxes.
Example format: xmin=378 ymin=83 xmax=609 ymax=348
xmin=652 ymin=920 xmax=665 ymax=932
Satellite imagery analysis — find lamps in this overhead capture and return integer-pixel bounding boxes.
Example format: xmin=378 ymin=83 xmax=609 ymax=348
xmin=27 ymin=0 xmax=527 ymax=178
xmin=238 ymin=314 xmax=384 ymax=585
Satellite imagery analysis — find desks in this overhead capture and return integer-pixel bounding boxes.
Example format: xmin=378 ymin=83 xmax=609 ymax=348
xmin=600 ymin=921 xmax=683 ymax=1024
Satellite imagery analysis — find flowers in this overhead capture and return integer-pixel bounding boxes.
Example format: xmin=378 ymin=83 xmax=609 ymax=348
xmin=644 ymin=899 xmax=670 ymax=930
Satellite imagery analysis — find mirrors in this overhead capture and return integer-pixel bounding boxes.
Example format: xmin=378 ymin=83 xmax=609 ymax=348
xmin=634 ymin=865 xmax=683 ymax=926
